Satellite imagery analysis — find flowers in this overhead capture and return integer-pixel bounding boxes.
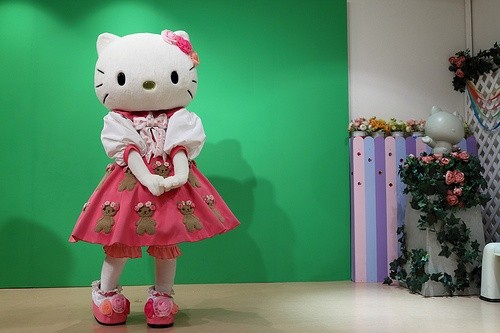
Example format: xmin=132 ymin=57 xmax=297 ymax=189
xmin=384 ymin=151 xmax=492 ymax=295
xmin=448 ymin=41 xmax=500 ymax=94
xmin=347 ymin=115 xmax=474 ymax=133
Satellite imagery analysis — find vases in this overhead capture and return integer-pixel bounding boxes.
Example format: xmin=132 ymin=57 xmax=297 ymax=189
xmin=353 ymin=131 xmax=364 ymax=137
xmin=392 ymin=131 xmax=405 ymax=138
xmin=372 ymin=132 xmax=385 ymax=138
xmin=412 ymin=132 xmax=424 ymax=138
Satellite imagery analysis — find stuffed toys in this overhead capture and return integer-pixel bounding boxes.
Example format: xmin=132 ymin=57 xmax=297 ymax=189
xmin=69 ymin=31 xmax=241 ymax=326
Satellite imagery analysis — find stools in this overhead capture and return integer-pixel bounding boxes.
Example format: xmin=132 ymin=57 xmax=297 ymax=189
xmin=479 ymin=242 xmax=500 ymax=302
